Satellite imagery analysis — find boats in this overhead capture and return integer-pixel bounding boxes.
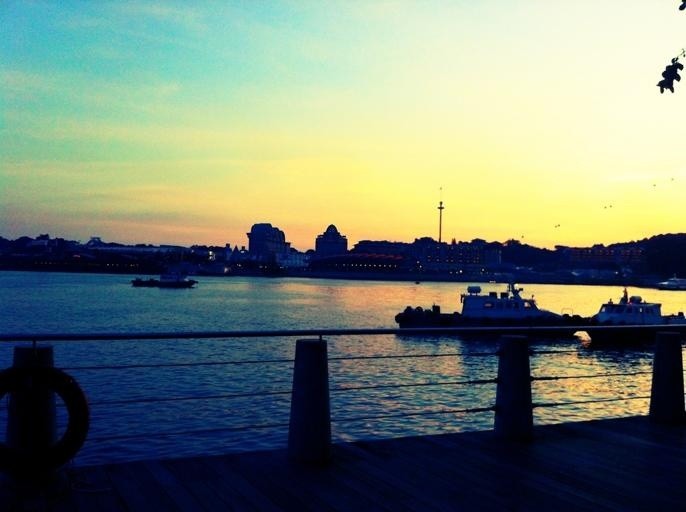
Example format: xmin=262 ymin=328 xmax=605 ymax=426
xmin=389 ymin=273 xmax=580 ymax=346
xmin=658 ymin=272 xmax=685 ymax=290
xmin=131 ymin=268 xmax=199 ymax=290
xmin=575 ymin=284 xmax=686 ymax=353
